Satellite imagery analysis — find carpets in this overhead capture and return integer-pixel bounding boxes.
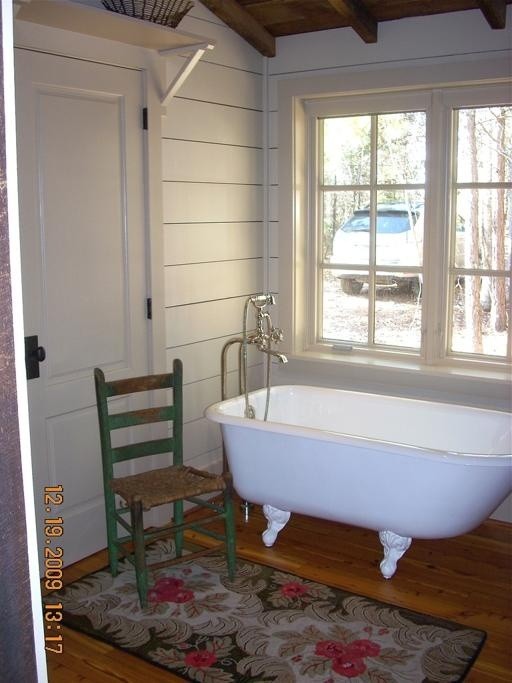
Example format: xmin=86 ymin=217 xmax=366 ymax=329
xmin=41 ymin=534 xmax=488 ymax=681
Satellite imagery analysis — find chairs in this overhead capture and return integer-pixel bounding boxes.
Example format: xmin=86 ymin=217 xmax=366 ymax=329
xmin=93 ymin=358 xmax=237 ymax=611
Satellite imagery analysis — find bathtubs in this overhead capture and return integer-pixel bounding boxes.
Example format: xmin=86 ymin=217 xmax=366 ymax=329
xmin=203 ymin=383 xmax=512 ymax=578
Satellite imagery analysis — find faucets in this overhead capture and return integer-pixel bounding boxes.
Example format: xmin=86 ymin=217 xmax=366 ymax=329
xmin=258 ymin=345 xmax=288 ymax=366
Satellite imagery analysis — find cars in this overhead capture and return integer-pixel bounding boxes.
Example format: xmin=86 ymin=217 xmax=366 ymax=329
xmin=332 ymin=201 xmax=467 ymax=294
